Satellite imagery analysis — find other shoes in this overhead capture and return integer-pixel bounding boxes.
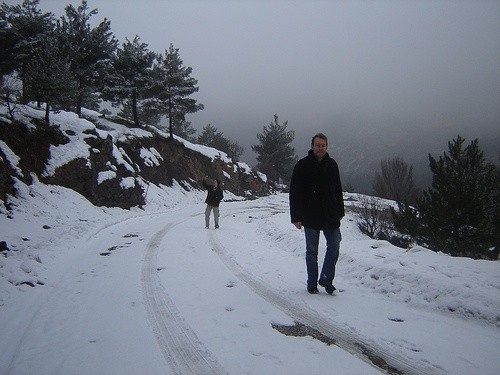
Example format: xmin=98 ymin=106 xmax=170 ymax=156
xmin=214 ymin=226 xmax=220 ymax=230
xmin=204 ymin=225 xmax=209 ymax=230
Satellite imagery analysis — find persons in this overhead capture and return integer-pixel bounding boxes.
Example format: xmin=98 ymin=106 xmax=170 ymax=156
xmin=203 ymin=176 xmax=224 ymax=229
xmin=289 ymin=133 xmax=345 ymax=295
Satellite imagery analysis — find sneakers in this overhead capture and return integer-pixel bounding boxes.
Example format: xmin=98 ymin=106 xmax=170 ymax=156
xmin=318 ymin=278 xmax=336 ymax=294
xmin=307 ymin=282 xmax=319 ymax=294
